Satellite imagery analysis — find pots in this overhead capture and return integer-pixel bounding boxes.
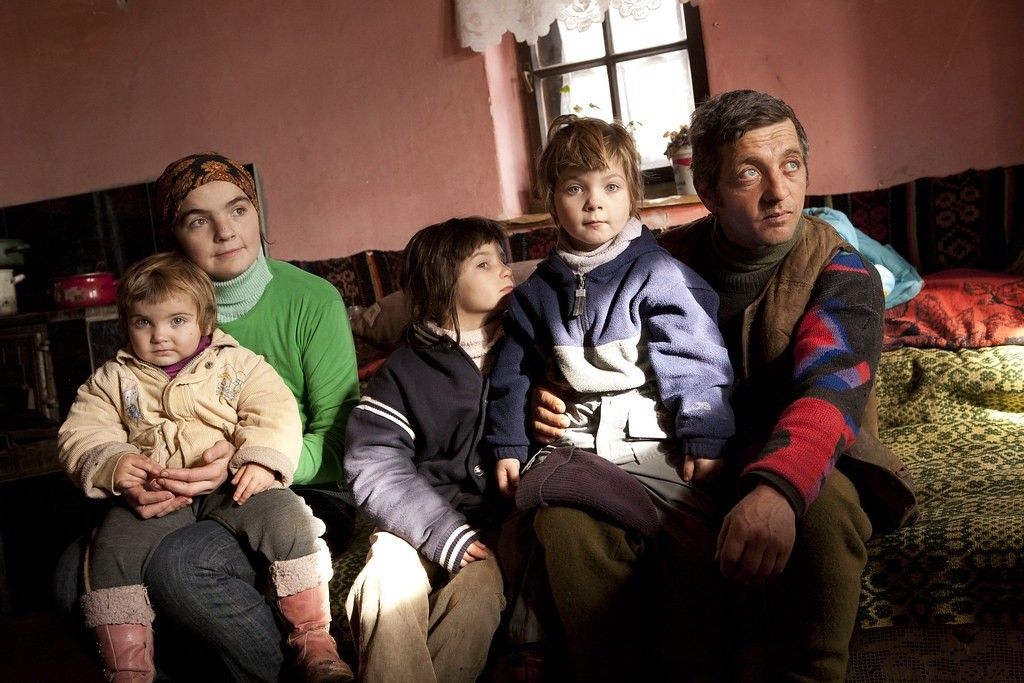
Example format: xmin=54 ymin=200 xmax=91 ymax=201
xmin=45 ymin=272 xmax=121 ymax=309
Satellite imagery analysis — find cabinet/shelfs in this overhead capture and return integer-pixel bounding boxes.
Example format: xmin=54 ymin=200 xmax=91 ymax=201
xmin=0 ymin=304 xmax=130 ymax=483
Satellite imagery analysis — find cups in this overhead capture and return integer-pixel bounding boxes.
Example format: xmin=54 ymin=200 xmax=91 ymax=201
xmin=671 ymin=151 xmax=698 ymax=196
xmin=0 ymin=269 xmax=24 ymax=314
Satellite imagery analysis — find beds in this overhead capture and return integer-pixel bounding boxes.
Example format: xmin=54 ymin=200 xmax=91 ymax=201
xmin=262 ymin=160 xmax=1024 ymax=683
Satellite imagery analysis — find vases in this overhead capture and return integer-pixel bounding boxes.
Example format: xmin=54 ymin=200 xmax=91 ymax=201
xmin=671 ymin=146 xmax=697 ymax=195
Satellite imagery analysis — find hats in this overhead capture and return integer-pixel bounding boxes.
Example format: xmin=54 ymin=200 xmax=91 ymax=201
xmin=515 ymin=446 xmax=658 ymax=541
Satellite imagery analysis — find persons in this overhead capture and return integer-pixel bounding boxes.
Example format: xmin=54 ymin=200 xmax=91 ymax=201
xmin=489 ymin=114 xmax=735 ymax=610
xmin=55 ymin=153 xmax=361 ymax=682
xmin=528 ymin=89 xmax=917 ymax=682
xmin=342 ymin=216 xmax=518 ymax=683
xmin=56 ymin=254 xmax=352 ymax=683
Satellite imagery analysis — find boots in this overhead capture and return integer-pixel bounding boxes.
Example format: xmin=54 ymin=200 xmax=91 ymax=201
xmin=269 ymin=547 xmax=354 ymax=683
xmin=80 ymin=582 xmax=155 ymax=683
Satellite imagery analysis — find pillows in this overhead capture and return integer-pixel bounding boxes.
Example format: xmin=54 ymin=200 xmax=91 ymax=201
xmin=352 ymin=260 xmax=550 ymax=355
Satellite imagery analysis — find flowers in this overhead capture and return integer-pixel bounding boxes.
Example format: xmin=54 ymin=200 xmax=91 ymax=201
xmin=663 ymin=124 xmax=699 ymax=158
